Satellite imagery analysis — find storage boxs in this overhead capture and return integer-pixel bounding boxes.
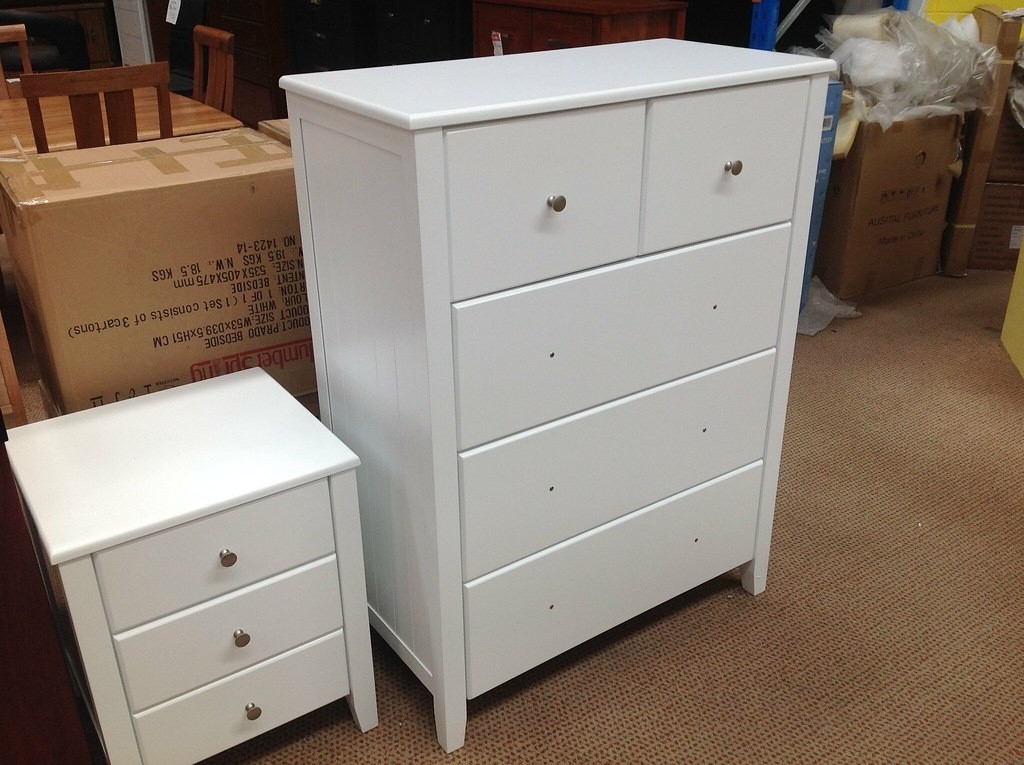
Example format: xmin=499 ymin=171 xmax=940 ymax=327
xmin=966 ymin=182 xmax=1024 ymax=271
xmin=813 ymin=114 xmax=958 ymax=299
xmin=939 ymin=4 xmax=1024 ymax=278
xmin=0 ymin=127 xmax=316 ymax=414
xmin=986 ymin=96 xmax=1024 ymax=182
xmin=800 ymin=79 xmax=842 ymax=312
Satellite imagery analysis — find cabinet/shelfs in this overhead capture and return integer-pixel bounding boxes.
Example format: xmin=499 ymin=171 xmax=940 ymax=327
xmin=4 ymin=3 xmax=114 ymax=79
xmin=281 ymin=37 xmax=836 ymax=753
xmin=148 ymin=0 xmax=292 ymax=130
xmin=472 ymin=0 xmax=688 ymax=57
xmin=4 ymin=367 xmax=379 ymax=765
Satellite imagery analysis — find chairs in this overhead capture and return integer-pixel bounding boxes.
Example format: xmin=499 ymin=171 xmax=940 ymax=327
xmin=0 ymin=24 xmax=33 ymax=101
xmin=191 ymin=25 xmax=236 ymax=119
xmin=18 ymin=61 xmax=173 ymax=155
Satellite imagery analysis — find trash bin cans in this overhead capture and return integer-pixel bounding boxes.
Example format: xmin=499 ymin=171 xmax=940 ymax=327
xmin=812 ymin=96 xmax=967 ymax=310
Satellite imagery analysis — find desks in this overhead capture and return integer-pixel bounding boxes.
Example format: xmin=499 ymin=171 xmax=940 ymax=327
xmin=0 ymin=86 xmax=245 ymax=158
